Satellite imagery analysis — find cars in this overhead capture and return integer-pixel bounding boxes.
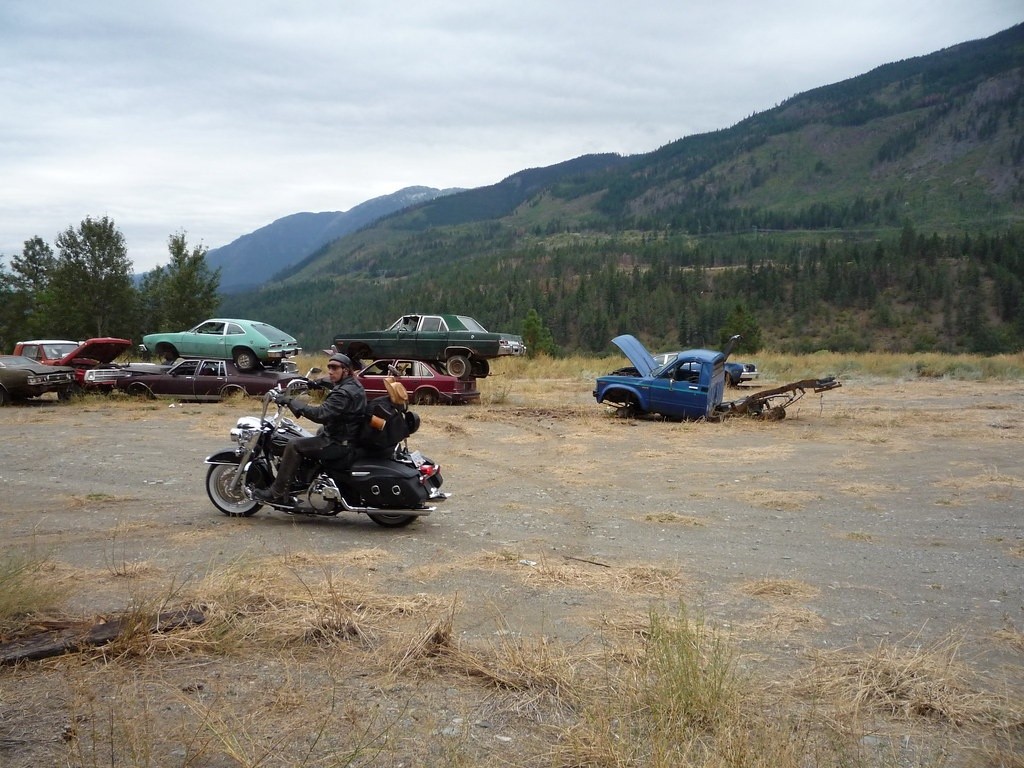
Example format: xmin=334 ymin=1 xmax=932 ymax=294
xmin=117 ymin=358 xmax=306 ymax=400
xmin=139 ymin=318 xmax=303 ymax=373
xmin=611 ymin=352 xmax=761 ymax=386
xmin=353 ymin=359 xmax=480 ymax=406
xmin=334 ymin=314 xmax=528 ymax=379
xmin=0 ymin=355 xmax=78 ymax=405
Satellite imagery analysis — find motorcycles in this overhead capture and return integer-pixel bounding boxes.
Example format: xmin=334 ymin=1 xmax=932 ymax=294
xmin=205 ymin=366 xmax=452 ymax=528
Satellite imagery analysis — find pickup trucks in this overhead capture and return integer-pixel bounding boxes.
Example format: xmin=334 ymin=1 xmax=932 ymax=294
xmin=593 ymin=332 xmax=842 ymax=421
xmin=15 ymin=336 xmax=133 ymax=394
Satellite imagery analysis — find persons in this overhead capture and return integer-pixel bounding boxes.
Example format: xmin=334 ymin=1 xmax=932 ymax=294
xmin=251 ymin=352 xmax=369 ymax=507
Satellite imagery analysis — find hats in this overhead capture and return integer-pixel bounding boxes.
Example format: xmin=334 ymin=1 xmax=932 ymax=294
xmin=328 ymin=360 xmax=344 ymax=366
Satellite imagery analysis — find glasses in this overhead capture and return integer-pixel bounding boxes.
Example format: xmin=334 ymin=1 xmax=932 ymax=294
xmin=327 ymin=364 xmax=344 ymax=370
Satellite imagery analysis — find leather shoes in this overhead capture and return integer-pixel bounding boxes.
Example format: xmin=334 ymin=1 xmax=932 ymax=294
xmin=253 ymin=486 xmax=288 ymax=504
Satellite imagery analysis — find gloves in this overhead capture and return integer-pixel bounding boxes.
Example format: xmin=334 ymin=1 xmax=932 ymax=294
xmin=313 ymin=377 xmax=323 ymax=390
xmin=275 ymin=393 xmax=289 ymax=405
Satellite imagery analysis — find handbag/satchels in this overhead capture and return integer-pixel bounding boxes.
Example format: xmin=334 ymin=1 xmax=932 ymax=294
xmin=359 ymin=395 xmax=407 ymax=445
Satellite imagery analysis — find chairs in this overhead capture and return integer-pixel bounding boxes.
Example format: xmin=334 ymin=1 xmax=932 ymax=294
xmin=204 ymin=367 xmax=218 ymax=376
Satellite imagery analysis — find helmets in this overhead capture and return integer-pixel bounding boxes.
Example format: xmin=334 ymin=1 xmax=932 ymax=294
xmin=328 ymin=353 xmax=352 ymax=368
xmin=405 ymin=411 xmax=420 ymax=434
